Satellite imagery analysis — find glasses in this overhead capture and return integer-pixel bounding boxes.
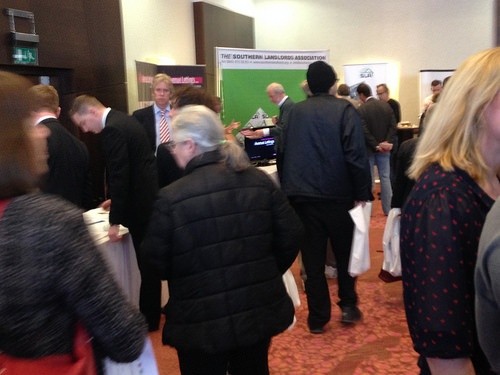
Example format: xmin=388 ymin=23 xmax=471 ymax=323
xmin=377 ymin=91 xmax=387 ymax=95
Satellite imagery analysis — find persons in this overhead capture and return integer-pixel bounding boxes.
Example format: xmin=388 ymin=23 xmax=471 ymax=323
xmin=0 ymin=70 xmax=146 ymax=375
xmin=0 ymin=46 xmax=500 ymax=375
xmin=276 ymin=59 xmax=374 ymax=333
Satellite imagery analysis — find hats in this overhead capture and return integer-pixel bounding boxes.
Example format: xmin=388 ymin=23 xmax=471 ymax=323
xmin=306 ymin=60 xmax=338 ymax=92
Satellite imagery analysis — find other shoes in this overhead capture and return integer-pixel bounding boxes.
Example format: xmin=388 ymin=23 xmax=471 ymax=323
xmin=341 ymin=304 xmax=363 ymax=324
xmin=308 ymin=322 xmax=323 ymax=334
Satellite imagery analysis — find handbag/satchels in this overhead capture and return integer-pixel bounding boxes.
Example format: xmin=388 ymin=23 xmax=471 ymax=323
xmin=347 ymin=201 xmax=371 ymax=278
xmin=376 ymin=207 xmax=404 ymax=284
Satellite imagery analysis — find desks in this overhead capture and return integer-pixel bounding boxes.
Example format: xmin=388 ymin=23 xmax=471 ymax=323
xmin=82 ymin=165 xmax=278 ymax=307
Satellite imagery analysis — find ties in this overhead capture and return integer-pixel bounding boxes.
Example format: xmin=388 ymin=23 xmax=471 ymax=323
xmin=160 ymin=108 xmax=171 ymax=144
